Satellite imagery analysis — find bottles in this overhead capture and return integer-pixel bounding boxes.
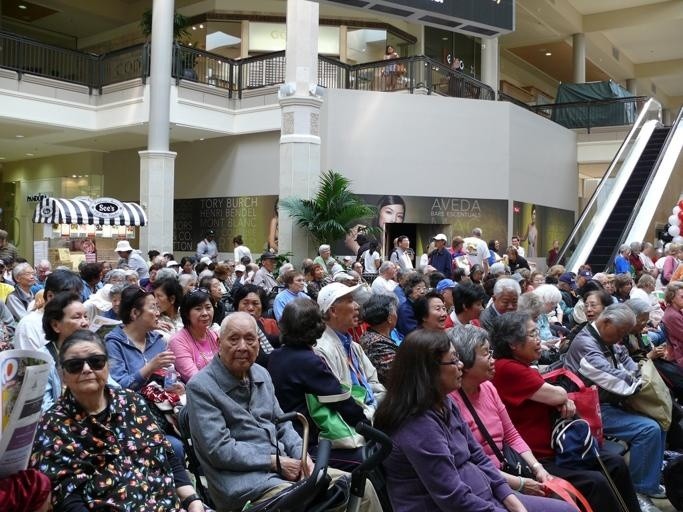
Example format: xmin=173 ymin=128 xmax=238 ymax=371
xmin=164 ymin=364 xmax=178 ymax=391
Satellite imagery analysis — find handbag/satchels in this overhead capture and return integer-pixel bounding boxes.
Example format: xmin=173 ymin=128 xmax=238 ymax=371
xmin=551 ymin=415 xmax=598 ymax=466
xmin=537 ymin=368 xmax=603 ymax=448
xmin=305 ymin=384 xmax=374 ymax=449
xmin=395 ymin=66 xmax=407 ymax=76
xmin=502 ymin=442 xmax=536 ymax=480
xmin=626 ymin=358 xmax=672 ymax=431
xmin=138 ymin=367 xmax=189 ymax=414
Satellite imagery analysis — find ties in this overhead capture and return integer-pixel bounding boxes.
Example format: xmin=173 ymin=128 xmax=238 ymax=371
xmin=343 ymin=342 xmax=363 ymax=385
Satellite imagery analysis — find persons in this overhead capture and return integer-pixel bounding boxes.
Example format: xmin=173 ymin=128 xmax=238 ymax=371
xmin=447 ymin=56 xmax=465 ymax=96
xmin=518 ymin=204 xmax=538 ymax=257
xmin=382 ymin=45 xmax=400 ymax=90
xmin=1 ymin=226 xmax=683 ymax=512
xmin=267 ymin=198 xmax=278 ymax=253
xmin=344 ymin=195 xmax=406 ymax=255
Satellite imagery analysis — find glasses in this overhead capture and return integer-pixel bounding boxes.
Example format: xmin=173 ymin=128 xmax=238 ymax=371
xmin=139 ymin=306 xmax=159 ymax=314
xmin=583 ymin=302 xmax=602 ymax=310
xmin=526 ymin=329 xmax=540 ymax=338
xmin=136 ymin=287 xmax=148 ymax=294
xmin=61 ymin=354 xmax=107 ymax=372
xmin=412 ymin=287 xmax=429 ymax=292
xmin=186 ymin=287 xmax=210 ymax=296
xmin=484 ymin=349 xmax=493 ymax=361
xmin=440 ymin=353 xmax=459 ymax=365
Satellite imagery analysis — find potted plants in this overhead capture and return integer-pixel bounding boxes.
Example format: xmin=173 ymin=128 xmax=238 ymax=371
xmin=138 ymin=7 xmax=192 ymax=80
xmin=181 ymin=41 xmax=199 ymax=79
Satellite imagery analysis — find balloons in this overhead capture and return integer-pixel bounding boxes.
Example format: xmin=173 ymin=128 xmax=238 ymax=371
xmin=655 ymin=198 xmax=683 ymax=258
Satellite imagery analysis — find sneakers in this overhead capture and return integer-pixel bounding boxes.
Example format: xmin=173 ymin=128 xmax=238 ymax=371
xmin=636 ymin=492 xmax=661 ymax=512
xmin=649 ymin=484 xmax=666 ymax=498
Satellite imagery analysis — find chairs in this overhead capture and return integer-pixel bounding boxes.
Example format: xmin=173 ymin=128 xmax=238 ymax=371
xmin=178 ymin=404 xmax=215 ymax=509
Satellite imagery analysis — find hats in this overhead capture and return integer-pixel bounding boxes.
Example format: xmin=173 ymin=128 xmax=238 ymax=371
xmin=580 ymin=271 xmax=592 ymax=279
xmin=208 ymin=229 xmax=215 ymax=236
xmin=88 ymin=284 xmax=114 ymax=311
xmin=316 ymin=281 xmax=366 ymax=312
xmin=0 ymin=259 xmax=5 ymax=266
xmin=342 ymin=255 xmax=351 ymax=261
xmin=234 ymin=264 xmax=245 ymax=272
xmin=331 ymin=264 xmax=346 ymax=276
xmin=432 ymin=234 xmax=447 ymax=242
xmin=436 ymin=279 xmax=457 ymax=293
xmin=113 ymin=240 xmax=132 ymax=252
xmin=354 ymin=234 xmax=364 ymax=241
xmin=199 ymin=257 xmax=213 ymax=265
xmin=166 ymin=261 xmax=180 ymax=268
xmin=559 ymin=272 xmax=576 ymax=288
xmin=261 ymin=252 xmax=280 ymax=261
